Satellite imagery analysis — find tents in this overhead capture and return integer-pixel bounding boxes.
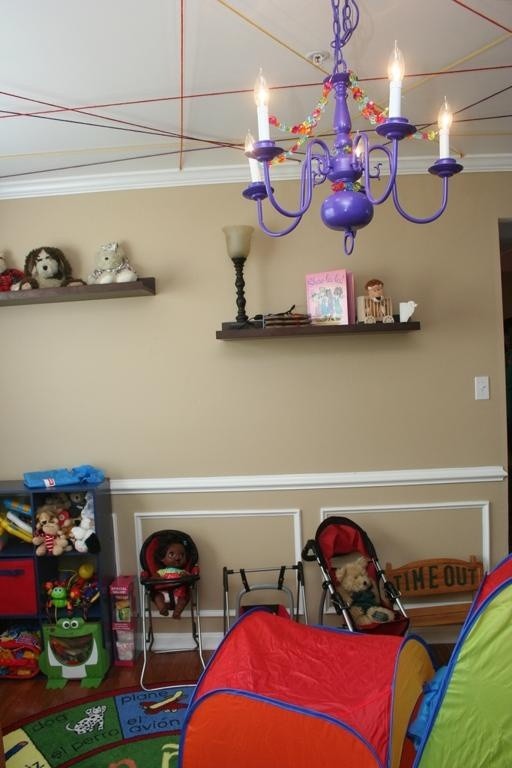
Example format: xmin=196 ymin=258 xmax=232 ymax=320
xmin=408 ymin=553 xmax=512 ymax=768
xmin=176 ymin=602 xmax=439 ymax=768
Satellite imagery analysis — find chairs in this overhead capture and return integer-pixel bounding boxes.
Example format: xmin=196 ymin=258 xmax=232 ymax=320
xmin=140 ymin=530 xmax=208 ymax=692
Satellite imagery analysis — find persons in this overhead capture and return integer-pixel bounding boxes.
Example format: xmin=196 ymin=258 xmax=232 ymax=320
xmin=146 ymin=542 xmax=191 ymax=619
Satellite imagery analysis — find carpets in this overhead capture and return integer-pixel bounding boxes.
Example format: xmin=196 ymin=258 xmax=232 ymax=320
xmin=1 ymin=679 xmax=199 ymax=768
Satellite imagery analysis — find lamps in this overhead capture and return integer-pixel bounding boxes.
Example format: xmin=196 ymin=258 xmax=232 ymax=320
xmin=244 ymin=0 xmax=463 ymax=255
xmin=221 ymin=224 xmax=263 ymax=330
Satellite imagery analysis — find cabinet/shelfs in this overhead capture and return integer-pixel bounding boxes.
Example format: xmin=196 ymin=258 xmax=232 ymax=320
xmin=0 ymin=488 xmax=108 ymax=653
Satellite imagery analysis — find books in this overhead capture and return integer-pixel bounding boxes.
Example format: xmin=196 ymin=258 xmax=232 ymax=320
xmin=306 ymin=269 xmax=355 ymax=326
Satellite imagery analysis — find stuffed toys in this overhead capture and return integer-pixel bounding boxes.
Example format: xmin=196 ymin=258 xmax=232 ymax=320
xmin=32 ymin=492 xmax=102 ymax=557
xmin=20 ymin=246 xmax=86 ymax=291
xmin=357 ymin=278 xmax=395 ymax=325
xmin=0 ymin=251 xmax=25 ymax=291
xmin=85 ymin=242 xmax=139 ymax=285
xmin=334 ymin=555 xmax=396 ymax=627
